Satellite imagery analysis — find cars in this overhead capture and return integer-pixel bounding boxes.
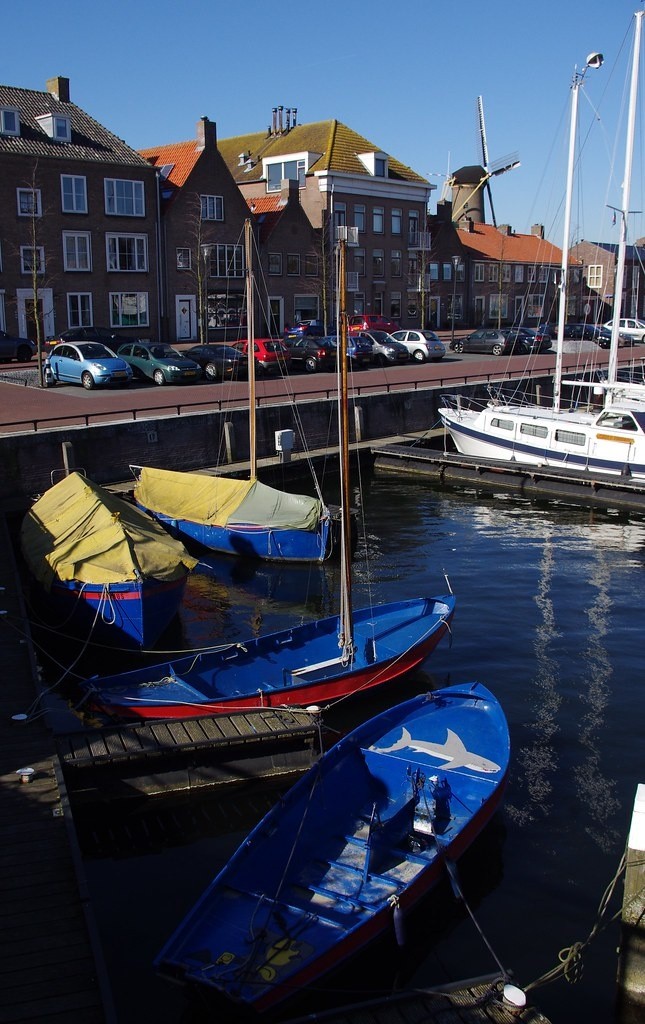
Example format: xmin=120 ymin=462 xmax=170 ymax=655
xmin=232 ymin=337 xmax=293 ymax=376
xmin=450 ymin=329 xmax=528 ymax=357
xmin=388 ymin=329 xmax=447 ymax=365
xmin=0 ymin=330 xmax=38 ymax=363
xmin=539 ymin=324 xmax=635 ymax=349
xmin=319 ymin=334 xmax=374 ymax=371
xmin=500 ymin=326 xmax=552 ymax=354
xmin=282 ymin=335 xmax=340 ymax=373
xmin=116 ymin=341 xmax=202 ymax=387
xmin=40 ymin=341 xmax=133 ymax=391
xmin=603 ymin=318 xmax=645 ymax=344
xmin=353 ymin=330 xmax=410 ymax=367
xmin=182 ymin=345 xmax=262 ymax=383
xmin=45 ymin=326 xmax=137 ymax=352
xmin=282 ymin=319 xmax=336 ymax=336
xmin=348 ymin=315 xmax=403 ymax=335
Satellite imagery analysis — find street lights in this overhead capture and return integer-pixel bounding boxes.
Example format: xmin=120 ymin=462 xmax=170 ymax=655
xmin=199 ymin=241 xmax=211 ymax=345
xmin=418 ymin=160 xmax=521 ymax=325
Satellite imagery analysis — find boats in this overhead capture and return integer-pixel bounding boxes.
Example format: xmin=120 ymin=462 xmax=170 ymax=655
xmin=282 ymin=970 xmax=558 ymax=1024
xmin=139 ymin=682 xmax=513 ymax=1002
xmin=34 ymin=474 xmax=201 ymax=646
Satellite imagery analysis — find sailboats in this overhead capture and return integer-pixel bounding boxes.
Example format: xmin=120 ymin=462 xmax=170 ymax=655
xmin=126 ymin=217 xmax=360 ymax=561
xmin=67 ymin=249 xmax=455 ymax=722
xmin=435 ymin=11 xmax=645 ymax=492
